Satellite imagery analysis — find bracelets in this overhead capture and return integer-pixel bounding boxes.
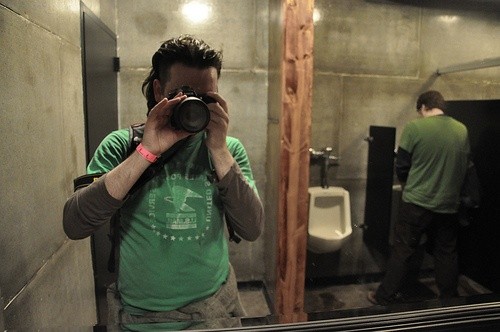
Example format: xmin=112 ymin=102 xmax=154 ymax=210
xmin=135 ymin=143 xmax=159 ymax=163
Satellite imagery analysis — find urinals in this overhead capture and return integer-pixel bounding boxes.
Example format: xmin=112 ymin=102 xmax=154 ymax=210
xmin=308 ymin=186 xmax=352 ymax=254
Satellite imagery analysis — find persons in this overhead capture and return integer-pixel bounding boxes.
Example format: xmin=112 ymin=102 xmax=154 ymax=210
xmin=63 ymin=37 xmax=264 ymax=324
xmin=367 ymin=91 xmax=471 ymax=307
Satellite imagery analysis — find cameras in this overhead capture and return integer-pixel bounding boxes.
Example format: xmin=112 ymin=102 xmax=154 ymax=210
xmin=167 ymin=86 xmax=216 ymax=134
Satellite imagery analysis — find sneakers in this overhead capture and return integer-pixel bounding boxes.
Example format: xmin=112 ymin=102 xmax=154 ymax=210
xmin=367 ymin=290 xmax=380 ymax=304
